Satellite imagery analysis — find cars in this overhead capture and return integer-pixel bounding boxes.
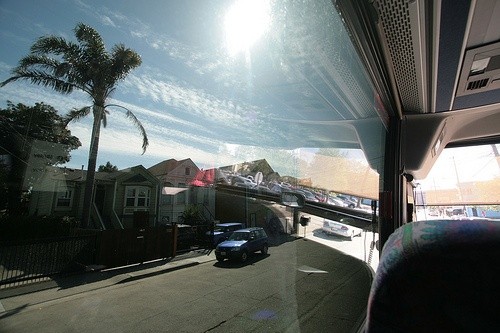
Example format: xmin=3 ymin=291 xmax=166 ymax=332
xmin=321 ymin=218 xmax=364 ymax=240
xmin=220 ymin=168 xmax=361 ymax=209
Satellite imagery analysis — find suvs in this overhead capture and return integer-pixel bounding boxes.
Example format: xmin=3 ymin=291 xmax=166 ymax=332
xmin=205 ymin=222 xmax=243 ymax=242
xmin=216 ymin=227 xmax=269 ymax=263
